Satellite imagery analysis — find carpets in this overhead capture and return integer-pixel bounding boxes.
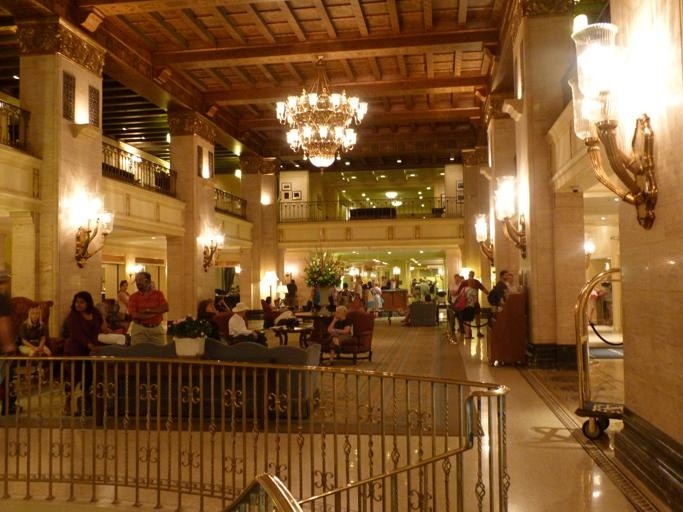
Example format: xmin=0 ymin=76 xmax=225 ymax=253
xmin=0 ymin=316 xmax=484 ymax=437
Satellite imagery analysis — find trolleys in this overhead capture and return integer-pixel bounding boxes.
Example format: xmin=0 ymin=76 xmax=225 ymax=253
xmin=575 ymin=268 xmax=623 ymax=439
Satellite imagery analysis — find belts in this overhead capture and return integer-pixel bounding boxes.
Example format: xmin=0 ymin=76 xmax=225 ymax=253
xmin=140 ymin=323 xmax=159 ymax=328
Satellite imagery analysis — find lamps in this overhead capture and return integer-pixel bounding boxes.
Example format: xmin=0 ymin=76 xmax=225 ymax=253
xmin=275 ymin=56 xmax=369 ymax=167
xmin=126 ymin=264 xmax=143 ymax=280
xmin=74 ymin=201 xmax=115 ymax=268
xmin=567 ymin=22 xmax=658 ymax=231
xmin=263 ymin=271 xmax=279 ymax=304
xmin=474 ymin=213 xmax=495 ymax=267
xmin=197 ymin=230 xmax=226 ymax=273
xmin=493 ymin=176 xmax=526 ymax=259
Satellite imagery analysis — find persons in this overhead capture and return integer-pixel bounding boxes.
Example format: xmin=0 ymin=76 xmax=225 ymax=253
xmin=327 ymin=281 xmax=382 ymax=365
xmin=448 ymin=270 xmax=513 ymax=339
xmin=21 ymin=307 xmax=51 ymax=368
xmin=1 ymin=273 xmax=20 ymax=415
xmin=207 ymin=295 xmax=230 ymax=314
xmin=61 ymin=272 xmax=169 ymax=383
xmin=229 ymin=303 xmax=265 ymax=343
xmin=402 ymin=278 xmax=435 ymax=327
xmin=287 ymin=280 xmax=298 ymax=305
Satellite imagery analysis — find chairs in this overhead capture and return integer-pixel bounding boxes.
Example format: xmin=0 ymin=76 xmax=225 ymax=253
xmin=198 ymin=289 xmax=375 ymax=364
xmin=409 ymin=304 xmax=436 ymax=328
xmin=10 ymin=296 xmax=63 ymax=383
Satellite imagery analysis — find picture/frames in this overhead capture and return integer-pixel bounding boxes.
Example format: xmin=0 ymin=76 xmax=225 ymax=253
xmin=281 ymin=182 xmax=302 ymax=200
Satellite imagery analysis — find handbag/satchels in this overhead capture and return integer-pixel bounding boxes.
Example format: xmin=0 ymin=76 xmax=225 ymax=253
xmin=453 ymin=293 xmax=466 ymax=311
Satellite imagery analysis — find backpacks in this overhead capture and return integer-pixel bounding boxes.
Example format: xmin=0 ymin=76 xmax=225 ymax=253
xmin=487 ymin=285 xmax=500 ymax=306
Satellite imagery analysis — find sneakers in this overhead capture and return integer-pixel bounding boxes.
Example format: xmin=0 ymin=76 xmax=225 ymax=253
xmin=1 ymin=401 xmax=24 ymax=416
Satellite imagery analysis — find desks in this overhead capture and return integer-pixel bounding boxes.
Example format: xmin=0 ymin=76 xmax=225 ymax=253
xmin=381 ymin=290 xmax=410 ymax=311
xmin=111 ymin=359 xmax=271 ymax=430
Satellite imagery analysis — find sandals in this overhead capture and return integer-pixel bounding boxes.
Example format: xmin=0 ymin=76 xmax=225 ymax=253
xmin=326 ymin=360 xmax=336 ymax=366
xmin=328 ymin=343 xmax=341 ymax=353
xmin=61 ymin=406 xmax=71 ymax=416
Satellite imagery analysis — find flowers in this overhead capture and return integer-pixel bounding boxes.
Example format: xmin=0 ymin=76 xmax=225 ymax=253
xmin=303 ymin=246 xmax=352 ymax=289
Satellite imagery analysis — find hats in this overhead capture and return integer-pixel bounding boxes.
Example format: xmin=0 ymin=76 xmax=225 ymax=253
xmin=232 ymin=303 xmax=251 ymax=313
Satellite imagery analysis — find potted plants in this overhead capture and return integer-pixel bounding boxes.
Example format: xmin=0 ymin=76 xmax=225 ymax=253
xmin=167 ymin=314 xmax=214 ymax=357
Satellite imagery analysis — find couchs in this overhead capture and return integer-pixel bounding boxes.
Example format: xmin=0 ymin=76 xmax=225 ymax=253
xmin=89 ymin=336 xmax=322 ymax=420
